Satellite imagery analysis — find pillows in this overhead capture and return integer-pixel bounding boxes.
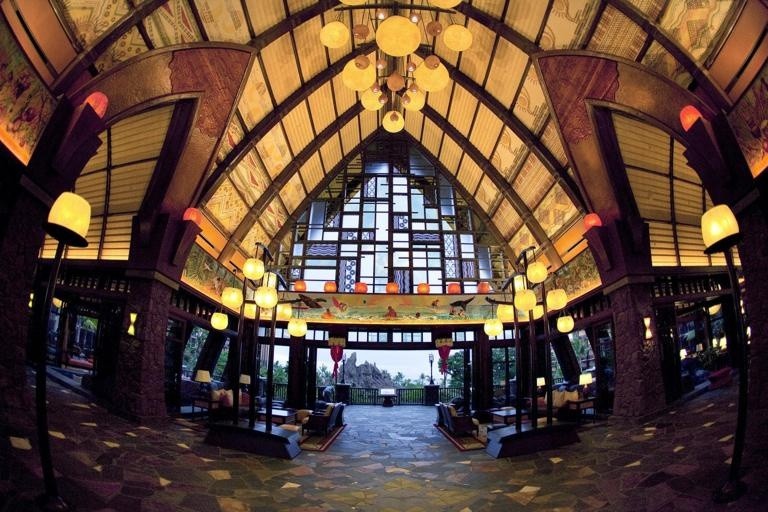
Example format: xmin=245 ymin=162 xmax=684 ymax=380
xmin=211 ymin=387 xmax=242 ymax=407
xmin=543 ymin=389 xmax=578 ymax=408
xmin=323 ymin=404 xmax=335 ymax=416
xmin=448 ymin=405 xmax=457 ymax=416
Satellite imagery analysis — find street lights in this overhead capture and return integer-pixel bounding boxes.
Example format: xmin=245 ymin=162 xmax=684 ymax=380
xmin=427 ymin=353 xmax=435 ymax=386
xmin=484 ymin=244 xmax=576 ymax=435
xmin=208 ymin=237 xmax=313 ymax=437
xmin=340 ymin=353 xmax=347 ymax=384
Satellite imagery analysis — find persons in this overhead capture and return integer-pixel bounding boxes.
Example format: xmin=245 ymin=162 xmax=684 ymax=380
xmin=450 ymin=306 xmax=456 ymax=315
xmin=384 ymin=306 xmax=396 ymax=317
xmin=415 ymin=312 xmax=420 ymax=319
xmin=432 ymin=299 xmax=439 ymax=307
xmin=458 ymin=308 xmax=464 ymax=318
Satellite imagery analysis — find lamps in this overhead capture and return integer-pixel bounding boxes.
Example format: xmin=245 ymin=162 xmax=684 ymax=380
xmin=184 ymin=206 xmax=203 ymax=225
xmin=239 ymin=373 xmax=251 ymax=391
xmin=316 ymin=0 xmax=473 ymax=134
xmin=195 ymin=370 xmax=211 ymax=390
xmin=582 ymin=212 xmax=603 ymax=229
xmin=211 ymin=246 xmax=308 ymax=337
xmin=328 ymin=332 xmax=347 ymax=383
xmin=536 ymin=376 xmax=546 ymax=391
xmin=480 ymin=248 xmax=578 ymax=337
xmin=432 ymin=331 xmax=454 ymax=374
xmin=286 ymin=280 xmax=490 ymax=294
xmin=579 ymin=373 xmax=592 ymax=398
xmin=83 ymin=90 xmax=108 ymax=119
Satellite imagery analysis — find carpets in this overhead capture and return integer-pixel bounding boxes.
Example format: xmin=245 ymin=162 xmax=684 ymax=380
xmin=172 ymin=410 xmax=348 ymax=453
xmin=433 ymin=419 xmax=607 ymax=451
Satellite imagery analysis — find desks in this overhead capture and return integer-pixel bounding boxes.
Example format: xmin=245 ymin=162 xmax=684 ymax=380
xmin=568 ymin=396 xmax=597 ymax=423
xmin=377 ymin=394 xmax=397 ymax=406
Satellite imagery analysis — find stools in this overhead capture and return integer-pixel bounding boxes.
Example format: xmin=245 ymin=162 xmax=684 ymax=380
xmin=297 ymin=409 xmax=314 ymax=421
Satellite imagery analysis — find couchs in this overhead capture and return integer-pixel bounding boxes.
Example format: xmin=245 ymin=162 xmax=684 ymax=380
xmin=529 ymin=388 xmax=580 ymax=415
xmin=440 ymin=401 xmax=480 ymax=437
xmin=192 ymin=385 xmax=249 ymax=418
xmin=301 ymin=401 xmax=344 ymax=436
